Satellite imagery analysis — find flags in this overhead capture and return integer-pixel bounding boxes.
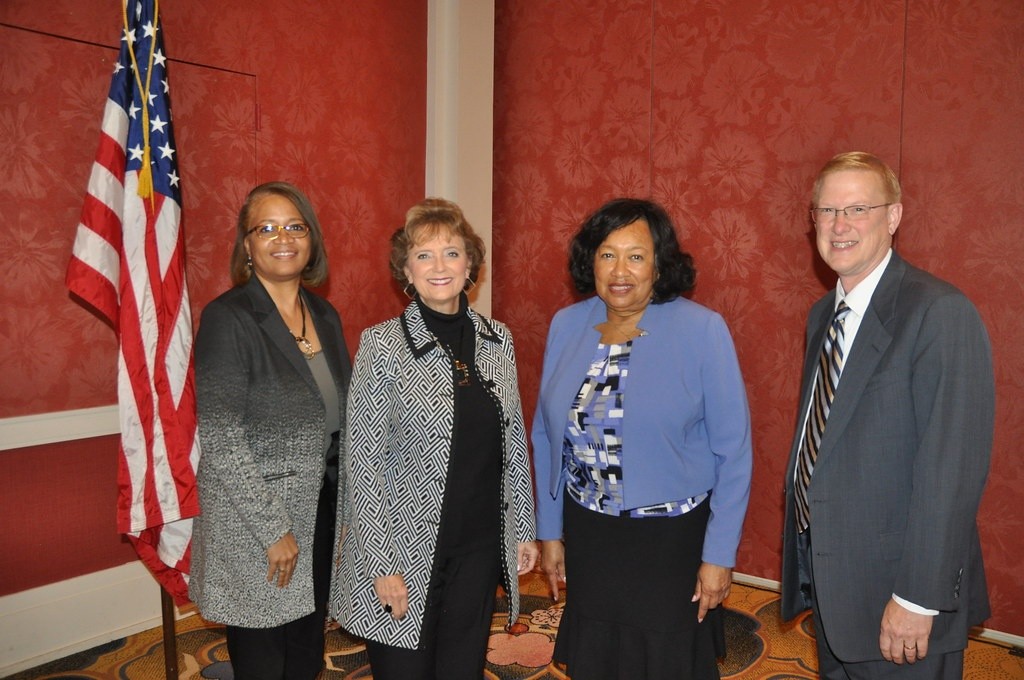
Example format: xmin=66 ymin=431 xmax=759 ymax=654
xmin=64 ymin=0 xmax=196 ymax=610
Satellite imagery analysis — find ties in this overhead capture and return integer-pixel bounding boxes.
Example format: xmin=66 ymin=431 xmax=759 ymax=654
xmin=794 ymin=300 xmax=852 ymax=533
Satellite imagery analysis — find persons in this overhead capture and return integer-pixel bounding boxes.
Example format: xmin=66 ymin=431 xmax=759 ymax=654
xmin=187 ymin=181 xmax=353 ymax=680
xmin=330 ymin=195 xmax=538 ymax=680
xmin=779 ymin=149 xmax=995 ymax=680
xmin=530 ymin=198 xmax=752 ymax=680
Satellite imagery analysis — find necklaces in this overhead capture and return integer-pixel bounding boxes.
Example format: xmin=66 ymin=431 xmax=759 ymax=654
xmin=290 ymin=292 xmax=315 ymax=360
xmin=607 ymin=321 xmax=637 ymax=341
xmin=432 ymin=326 xmax=473 ymax=387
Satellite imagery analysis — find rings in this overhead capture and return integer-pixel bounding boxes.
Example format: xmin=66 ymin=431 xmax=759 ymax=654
xmin=904 ymin=646 xmax=916 ymax=650
xmin=287 ymin=569 xmax=294 ymax=572
xmin=279 ymin=568 xmax=286 ymax=572
xmin=385 ymin=604 xmax=392 ymax=613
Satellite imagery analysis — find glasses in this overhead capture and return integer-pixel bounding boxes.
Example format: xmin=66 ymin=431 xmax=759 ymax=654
xmin=809 ymin=203 xmax=891 ymax=223
xmin=248 ymin=222 xmax=310 ymax=241
